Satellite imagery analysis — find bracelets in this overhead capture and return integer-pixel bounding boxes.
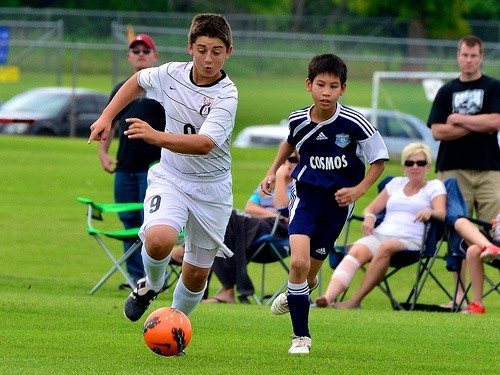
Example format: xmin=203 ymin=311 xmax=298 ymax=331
xmin=364 ymin=213 xmax=377 ymax=222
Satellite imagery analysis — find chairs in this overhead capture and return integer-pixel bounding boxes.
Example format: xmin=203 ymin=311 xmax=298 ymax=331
xmin=246 ymin=199 xmax=356 ymax=306
xmin=330 ymin=176 xmax=500 ymax=313
xmin=77 ymin=161 xmax=190 ymax=297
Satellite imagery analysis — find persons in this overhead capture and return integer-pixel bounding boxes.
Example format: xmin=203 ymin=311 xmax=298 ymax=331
xmin=257 ymin=54 xmax=389 ymax=354
xmin=87 ymin=12 xmax=239 ymax=331
xmin=426 ymin=34 xmax=500 ymax=315
xmin=96 ymin=32 xmax=171 ymax=292
xmin=172 ymin=143 xmax=326 ymax=305
xmin=455 ymin=211 xmax=500 ymax=315
xmin=315 ymin=141 xmax=450 ymax=308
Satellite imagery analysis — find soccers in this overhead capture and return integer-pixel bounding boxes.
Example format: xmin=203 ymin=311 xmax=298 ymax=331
xmin=143 ymin=307 xmax=193 ymax=357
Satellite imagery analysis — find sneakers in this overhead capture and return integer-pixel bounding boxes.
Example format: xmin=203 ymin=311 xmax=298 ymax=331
xmin=123 ymin=277 xmax=166 ymax=322
xmin=461 ymin=301 xmax=485 ymax=314
xmin=271 ymin=290 xmax=290 ymax=315
xmin=176 ymin=349 xmax=186 ymax=356
xmin=480 ymin=244 xmax=500 ymax=263
xmin=288 ymin=333 xmax=312 ymax=354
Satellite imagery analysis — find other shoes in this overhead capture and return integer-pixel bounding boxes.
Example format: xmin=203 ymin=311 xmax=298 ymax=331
xmin=119 ymin=283 xmax=138 ymax=292
xmin=439 ymin=300 xmax=468 ymax=311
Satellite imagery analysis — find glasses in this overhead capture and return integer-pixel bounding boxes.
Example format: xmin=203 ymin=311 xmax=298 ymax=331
xmin=288 ymin=157 xmax=299 ymax=163
xmin=131 ymin=46 xmax=153 ymax=54
xmin=405 ymin=160 xmax=427 ymax=166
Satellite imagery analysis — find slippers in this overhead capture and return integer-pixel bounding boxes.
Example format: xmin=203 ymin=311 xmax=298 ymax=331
xmin=209 ymin=297 xmax=227 ymax=303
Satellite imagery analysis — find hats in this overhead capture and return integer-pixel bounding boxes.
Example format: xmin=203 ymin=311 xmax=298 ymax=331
xmin=129 ymin=33 xmax=155 ymax=51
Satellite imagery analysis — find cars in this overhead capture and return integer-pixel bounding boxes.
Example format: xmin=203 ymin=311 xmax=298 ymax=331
xmin=348 ymin=106 xmax=441 ymax=161
xmin=234 ymin=126 xmax=291 ymax=146
xmin=0 ymin=87 xmax=109 ymax=136
